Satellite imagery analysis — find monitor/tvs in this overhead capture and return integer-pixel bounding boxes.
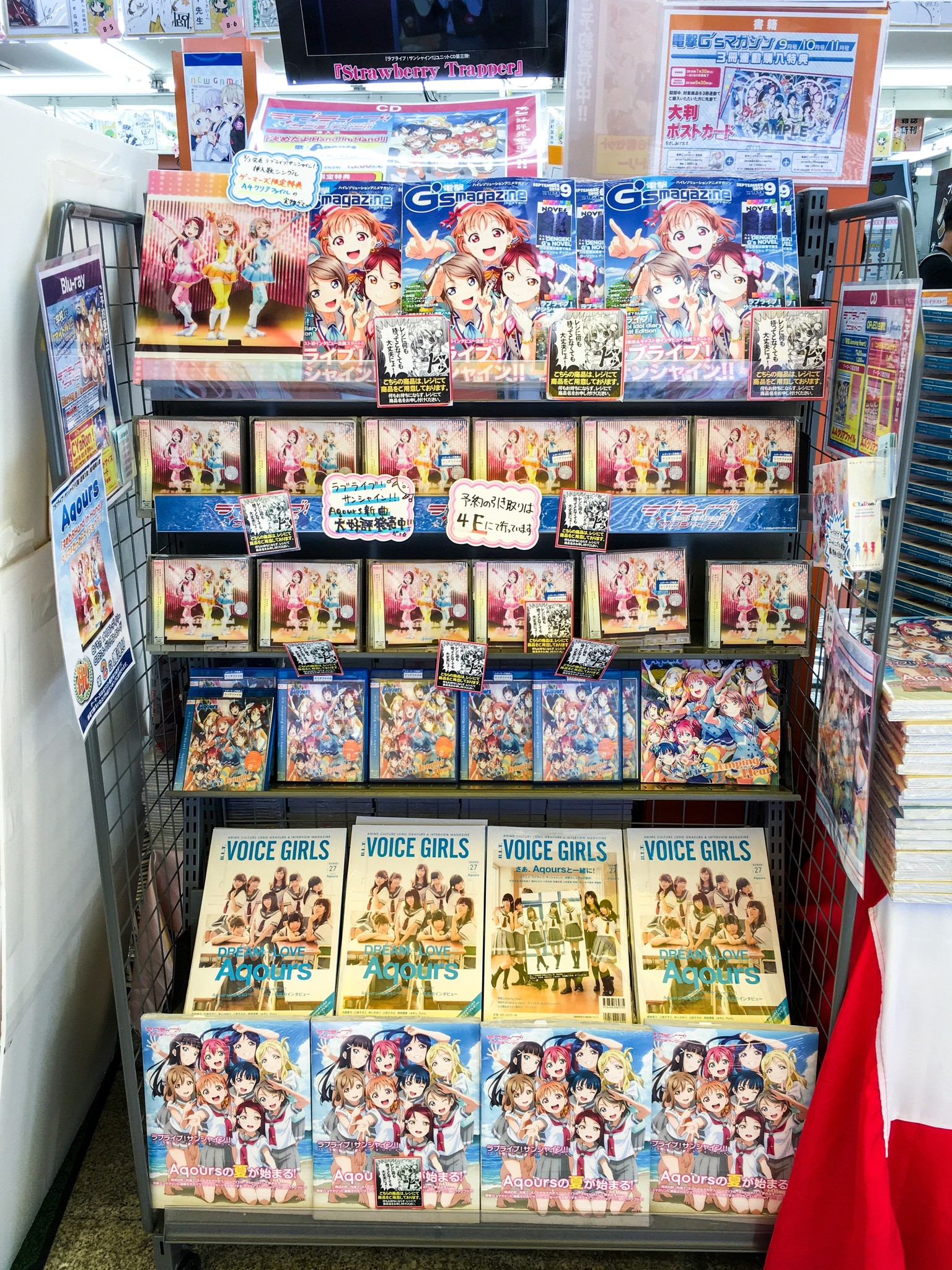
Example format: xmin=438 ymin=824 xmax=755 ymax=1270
xmin=275 ymin=0 xmax=567 ymax=85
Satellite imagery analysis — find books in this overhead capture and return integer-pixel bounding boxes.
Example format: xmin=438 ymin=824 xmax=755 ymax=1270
xmin=336 ymin=816 xmax=488 ymax=1020
xmin=140 ymin=1013 xmax=819 ymax=1228
xmin=134 ymin=167 xmax=798 ymax=791
xmin=841 ymin=616 xmax=952 ymax=904
xmin=484 ymin=825 xmax=632 ymax=1024
xmin=624 ymin=828 xmax=790 ymax=1024
xmin=183 ymin=827 xmax=347 ymax=1016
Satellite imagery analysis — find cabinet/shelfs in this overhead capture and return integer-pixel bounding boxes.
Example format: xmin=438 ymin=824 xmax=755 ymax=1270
xmin=34 ymin=186 xmax=929 ymax=1270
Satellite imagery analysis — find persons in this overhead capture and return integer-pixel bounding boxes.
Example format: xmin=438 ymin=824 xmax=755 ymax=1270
xmin=642 ymin=866 xmax=777 ymax=974
xmin=491 ymin=888 xmax=620 ymax=996
xmin=204 ymin=867 xmax=333 ymax=1001
xmin=918 ymin=200 xmax=952 ymax=289
xmin=350 ymin=863 xmax=476 ymax=987
